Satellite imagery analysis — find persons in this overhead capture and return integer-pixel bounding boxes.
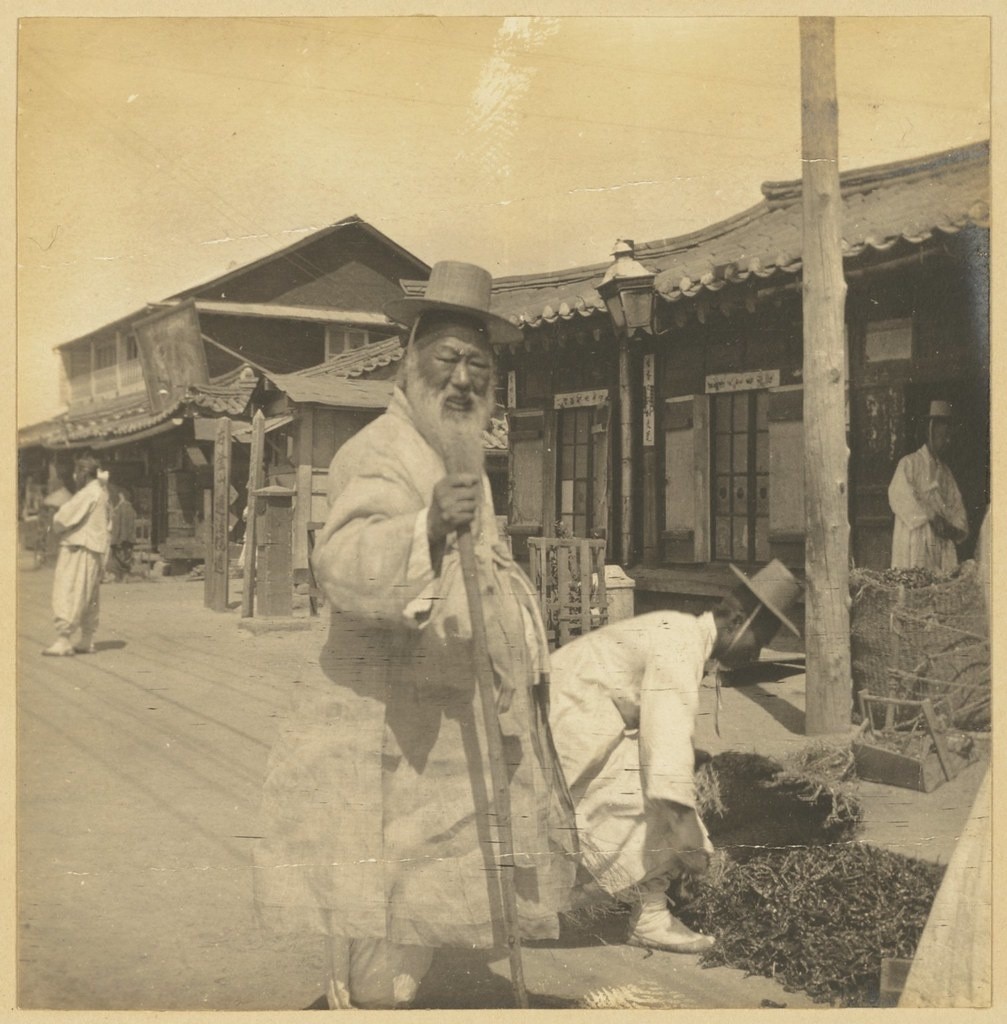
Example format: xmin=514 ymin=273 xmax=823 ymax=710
xmin=309 ymin=310 xmax=551 ymax=1010
xmin=888 ymin=415 xmax=968 ymax=573
xmin=42 ymin=452 xmax=138 ymax=656
xmin=551 ymin=582 xmax=782 ymax=953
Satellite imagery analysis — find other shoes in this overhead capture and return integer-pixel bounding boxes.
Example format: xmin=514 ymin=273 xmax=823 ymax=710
xmin=621 ymin=922 xmax=717 ymax=955
xmin=42 ymin=639 xmax=73 ymax=656
xmin=416 ymin=968 xmax=511 ymax=1003
xmin=72 ymin=639 xmax=95 ymax=653
xmin=102 ymin=573 xmax=123 ymax=584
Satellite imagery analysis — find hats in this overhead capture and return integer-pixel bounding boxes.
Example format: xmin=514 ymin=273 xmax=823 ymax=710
xmin=918 ymin=401 xmax=962 ymax=426
xmin=381 ymin=261 xmax=525 ymax=344
xmin=71 ymin=451 xmax=100 ymax=467
xmin=730 ymin=557 xmax=803 ymax=639
